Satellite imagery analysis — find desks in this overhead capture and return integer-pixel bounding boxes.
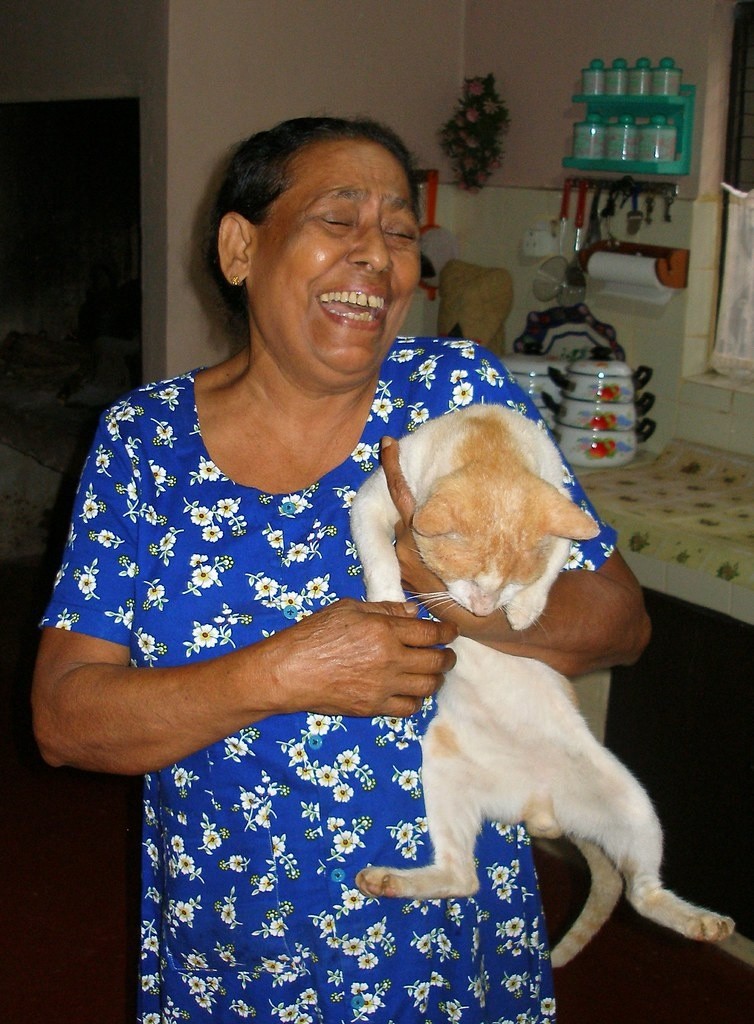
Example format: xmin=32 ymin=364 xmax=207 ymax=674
xmin=562 ymin=442 xmax=754 ymax=972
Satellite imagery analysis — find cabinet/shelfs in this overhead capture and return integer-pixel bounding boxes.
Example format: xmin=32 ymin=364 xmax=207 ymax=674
xmin=562 ymin=84 xmax=696 ymax=176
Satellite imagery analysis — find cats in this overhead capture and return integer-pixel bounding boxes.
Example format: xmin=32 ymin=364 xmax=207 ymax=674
xmin=350 ymin=405 xmax=736 ymax=970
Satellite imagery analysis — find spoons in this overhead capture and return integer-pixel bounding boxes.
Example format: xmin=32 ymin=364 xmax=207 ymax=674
xmin=627 ymin=181 xmax=643 ymax=224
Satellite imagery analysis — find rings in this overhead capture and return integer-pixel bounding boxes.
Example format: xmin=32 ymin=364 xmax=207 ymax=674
xmin=393 ymin=540 xmax=397 ymax=547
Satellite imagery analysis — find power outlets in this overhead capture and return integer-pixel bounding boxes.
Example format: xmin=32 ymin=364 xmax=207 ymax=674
xmin=522 ymin=230 xmax=551 ymax=257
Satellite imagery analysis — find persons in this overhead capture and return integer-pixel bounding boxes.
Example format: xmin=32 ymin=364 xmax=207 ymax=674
xmin=31 ymin=117 xmax=652 ymax=1024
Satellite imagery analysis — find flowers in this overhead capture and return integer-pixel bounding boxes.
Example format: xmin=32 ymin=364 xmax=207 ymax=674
xmin=438 ymin=71 xmax=512 ymax=196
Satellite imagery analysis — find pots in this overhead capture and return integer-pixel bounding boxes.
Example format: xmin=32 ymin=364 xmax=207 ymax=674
xmin=498 ymin=343 xmax=657 ymax=468
